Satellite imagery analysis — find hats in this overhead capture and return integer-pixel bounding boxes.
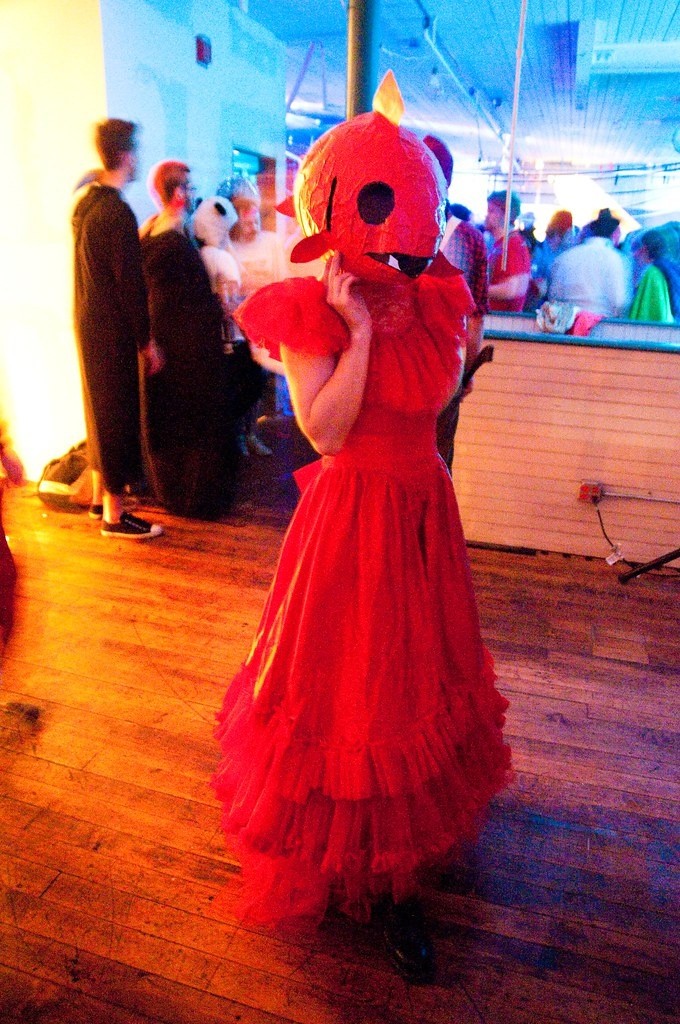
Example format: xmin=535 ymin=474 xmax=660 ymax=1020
xmin=488 ymin=190 xmax=522 ymax=220
xmin=589 ymin=208 xmax=620 ymax=230
xmin=547 ymin=211 xmax=573 ymax=234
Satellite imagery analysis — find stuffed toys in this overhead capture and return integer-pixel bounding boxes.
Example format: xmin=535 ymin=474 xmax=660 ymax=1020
xmin=190 ymin=197 xmax=243 ymax=323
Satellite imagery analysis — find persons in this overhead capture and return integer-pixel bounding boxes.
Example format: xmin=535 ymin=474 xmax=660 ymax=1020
xmin=222 ymin=177 xmax=680 ymax=514
xmin=208 ymin=109 xmax=512 ymax=920
xmin=69 ymin=118 xmax=165 ymax=543
xmin=131 ymin=159 xmax=226 ymax=519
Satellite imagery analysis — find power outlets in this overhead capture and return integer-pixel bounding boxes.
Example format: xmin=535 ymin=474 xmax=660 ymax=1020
xmin=577 ymin=480 xmax=602 ymax=504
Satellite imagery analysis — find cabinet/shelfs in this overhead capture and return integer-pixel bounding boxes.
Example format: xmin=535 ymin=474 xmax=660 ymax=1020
xmin=452 ymin=309 xmax=680 ymax=570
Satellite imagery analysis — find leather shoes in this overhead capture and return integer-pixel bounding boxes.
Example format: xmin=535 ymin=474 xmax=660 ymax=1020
xmin=432 ymin=868 xmax=479 ymax=895
xmin=382 ymin=897 xmax=438 ymax=984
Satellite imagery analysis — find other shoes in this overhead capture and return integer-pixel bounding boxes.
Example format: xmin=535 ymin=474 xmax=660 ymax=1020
xmin=100 ymin=512 xmax=163 ymax=538
xmin=88 ymin=503 xmax=103 ymax=519
